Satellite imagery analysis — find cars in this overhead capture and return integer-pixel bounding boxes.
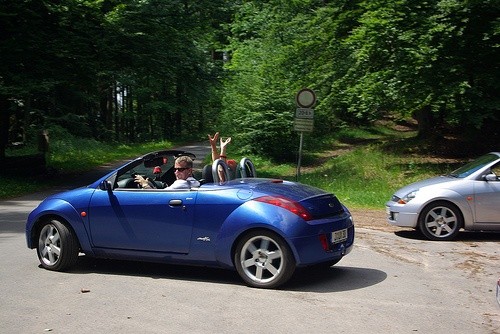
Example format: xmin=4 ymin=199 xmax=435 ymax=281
xmin=390 ymin=153 xmax=500 ymax=241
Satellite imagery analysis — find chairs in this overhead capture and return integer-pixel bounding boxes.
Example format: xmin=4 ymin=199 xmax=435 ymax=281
xmin=199 ymin=164 xmax=214 ymax=185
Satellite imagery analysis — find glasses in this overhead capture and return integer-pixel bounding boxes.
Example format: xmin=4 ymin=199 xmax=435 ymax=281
xmin=173 ymin=167 xmax=190 ymax=172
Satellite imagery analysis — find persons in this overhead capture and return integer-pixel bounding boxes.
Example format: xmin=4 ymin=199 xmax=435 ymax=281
xmin=208 ymin=131 xmax=237 ymax=186
xmin=134 ymin=156 xmax=201 ymax=190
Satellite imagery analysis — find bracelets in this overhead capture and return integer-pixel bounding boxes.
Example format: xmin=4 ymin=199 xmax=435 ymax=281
xmin=219 ymin=155 xmax=228 ymax=159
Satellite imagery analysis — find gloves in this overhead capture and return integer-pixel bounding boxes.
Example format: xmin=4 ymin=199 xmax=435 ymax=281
xmin=134 ymin=175 xmax=149 ymax=187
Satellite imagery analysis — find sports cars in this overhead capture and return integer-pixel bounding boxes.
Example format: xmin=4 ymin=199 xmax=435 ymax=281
xmin=25 ymin=149 xmax=355 ymax=288
